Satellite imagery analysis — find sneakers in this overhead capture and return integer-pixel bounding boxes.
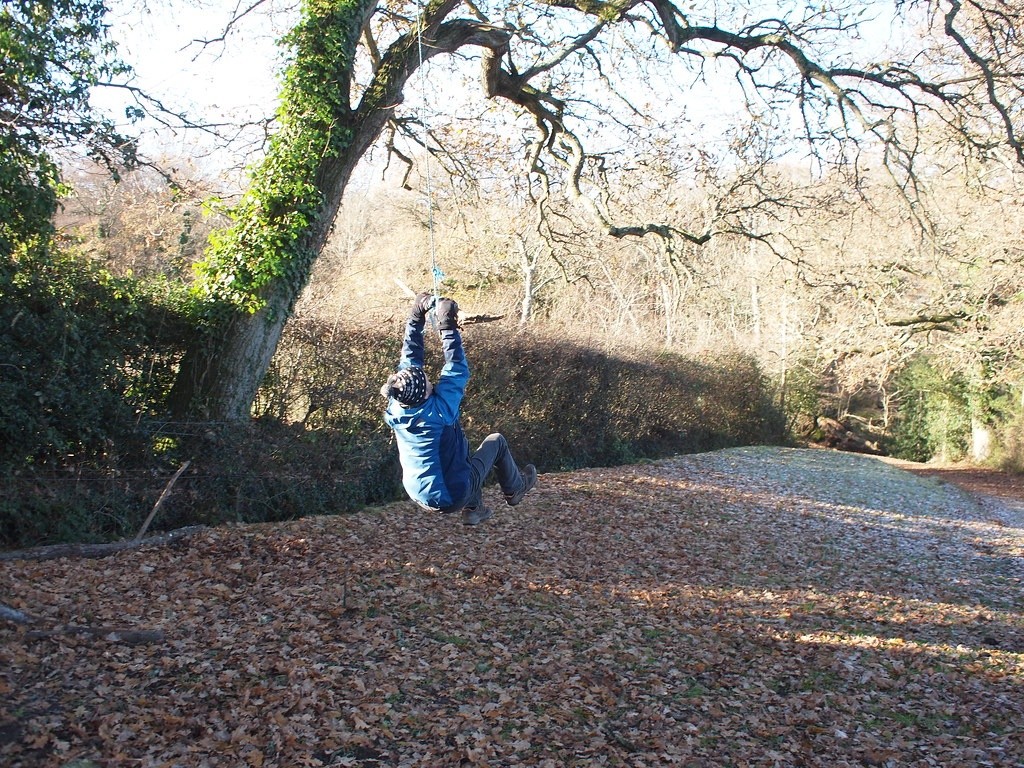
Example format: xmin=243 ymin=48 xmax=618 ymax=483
xmin=462 ymin=505 xmax=492 ymax=525
xmin=504 ymin=464 xmax=537 ymax=506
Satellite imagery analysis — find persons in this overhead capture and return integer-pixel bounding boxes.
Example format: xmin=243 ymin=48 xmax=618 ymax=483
xmin=380 ymin=292 xmax=537 ymax=525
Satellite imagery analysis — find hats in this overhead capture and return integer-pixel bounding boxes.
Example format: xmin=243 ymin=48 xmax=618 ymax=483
xmin=379 ymin=368 xmax=426 ymax=405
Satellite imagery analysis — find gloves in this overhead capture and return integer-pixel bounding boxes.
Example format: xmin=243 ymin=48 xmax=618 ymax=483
xmin=410 ymin=292 xmax=435 ymax=322
xmin=436 ymin=297 xmax=458 ymax=330
